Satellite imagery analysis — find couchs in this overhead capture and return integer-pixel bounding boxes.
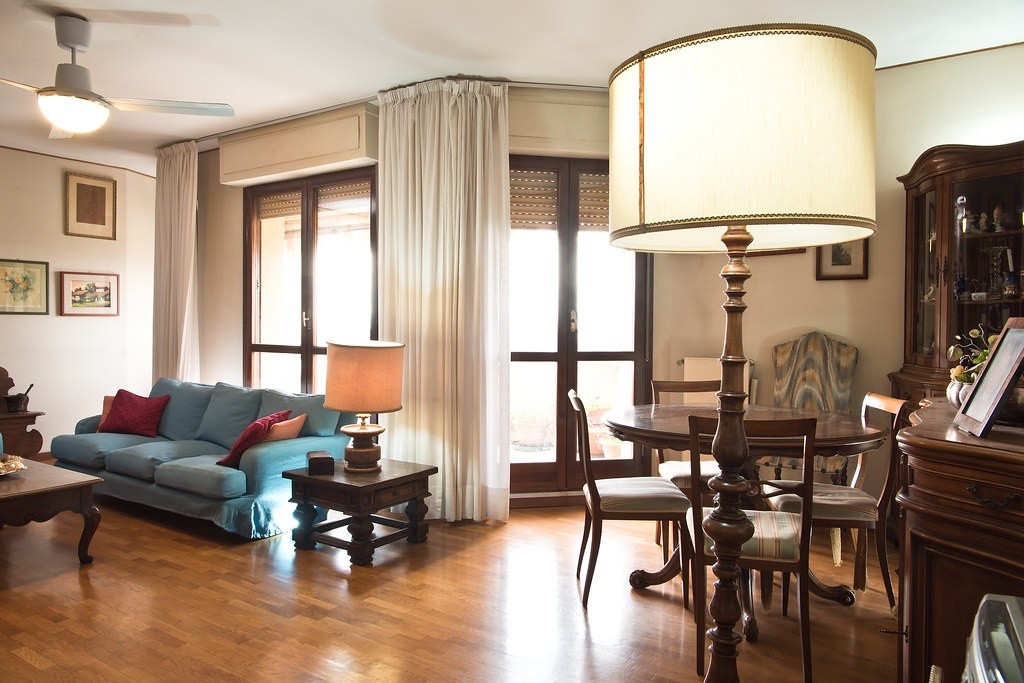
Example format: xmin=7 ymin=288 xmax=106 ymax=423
xmin=51 ymin=377 xmax=358 ymax=538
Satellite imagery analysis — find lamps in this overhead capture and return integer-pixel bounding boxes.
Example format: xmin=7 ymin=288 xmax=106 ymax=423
xmin=608 ymin=22 xmax=877 ymax=683
xmin=36 ymin=88 xmax=111 ymax=134
xmin=323 ymin=340 xmax=406 ymax=473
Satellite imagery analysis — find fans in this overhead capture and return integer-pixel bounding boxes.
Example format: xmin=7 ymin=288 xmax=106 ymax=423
xmin=0 ymin=16 xmax=235 ymax=139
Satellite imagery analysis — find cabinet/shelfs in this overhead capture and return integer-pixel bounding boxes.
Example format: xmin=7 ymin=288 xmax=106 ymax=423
xmin=887 ymin=371 xmax=1024 ymax=683
xmin=897 ymin=137 xmax=1024 ymax=375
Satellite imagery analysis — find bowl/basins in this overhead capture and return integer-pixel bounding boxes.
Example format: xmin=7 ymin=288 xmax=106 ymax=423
xmin=971 ymin=293 xmax=988 ymax=301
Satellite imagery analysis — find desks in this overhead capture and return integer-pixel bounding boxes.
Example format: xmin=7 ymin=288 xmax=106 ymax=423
xmin=0 ymin=366 xmax=46 ymax=459
xmin=0 ymin=454 xmax=104 ymax=564
xmin=600 ymin=402 xmax=890 ymax=605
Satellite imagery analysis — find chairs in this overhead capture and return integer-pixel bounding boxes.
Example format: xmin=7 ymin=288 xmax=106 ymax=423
xmin=687 ymin=415 xmax=818 ymax=683
xmin=755 ymin=331 xmax=858 ymax=567
xmin=650 ymin=380 xmax=726 ymax=566
xmin=760 ymin=391 xmax=909 ymax=616
xmin=568 ymin=388 xmax=690 ymax=610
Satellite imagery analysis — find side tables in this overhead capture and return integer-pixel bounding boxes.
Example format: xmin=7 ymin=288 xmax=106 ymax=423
xmin=282 ymin=458 xmax=438 ymax=566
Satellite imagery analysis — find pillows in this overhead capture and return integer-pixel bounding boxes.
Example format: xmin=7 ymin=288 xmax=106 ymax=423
xmin=95 ymin=395 xmax=116 ymax=433
xmin=215 ymin=409 xmax=293 ymax=465
xmin=98 ymin=388 xmax=170 ymax=438
xmin=261 ymin=413 xmax=307 ymax=442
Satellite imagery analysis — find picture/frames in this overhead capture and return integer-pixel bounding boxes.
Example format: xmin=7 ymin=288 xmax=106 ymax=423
xmin=61 ymin=271 xmax=120 ymax=316
xmin=953 ymin=317 xmax=1024 ymax=437
xmin=0 ymin=258 xmax=49 ymax=315
xmin=65 ymin=171 xmax=117 ymax=240
xmin=815 ymin=238 xmax=869 ymax=281
